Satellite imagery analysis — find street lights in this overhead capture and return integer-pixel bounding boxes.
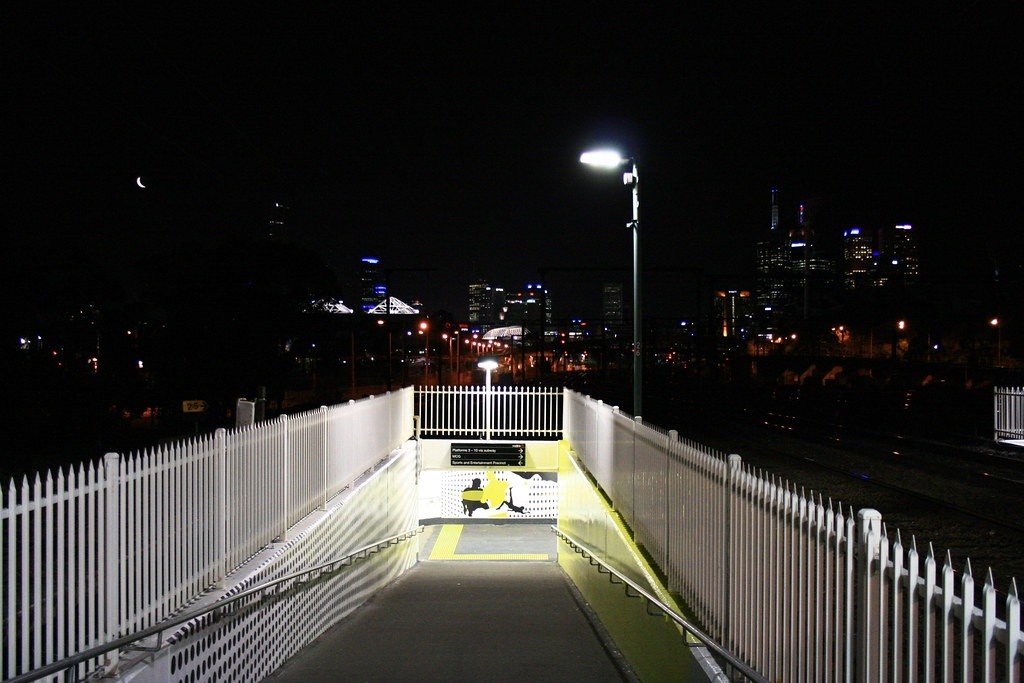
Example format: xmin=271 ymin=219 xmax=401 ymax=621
xmin=453 ymin=330 xmax=460 ymax=386
xmin=420 ymin=320 xmax=428 ymax=385
xmin=581 ymin=148 xmax=642 ymax=419
xmin=991 ymin=314 xmax=1001 ymax=368
xmin=378 ymin=319 xmax=392 ymax=378
